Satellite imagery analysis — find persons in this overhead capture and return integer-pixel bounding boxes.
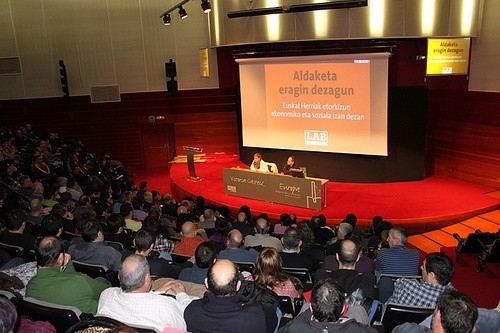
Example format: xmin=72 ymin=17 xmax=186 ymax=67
xmin=281 ymin=156 xmax=300 ymax=176
xmin=250 ymin=153 xmax=269 ymax=172
xmin=0 ymin=189 xmax=484 ymax=333
xmin=0 ymin=124 xmax=136 ymax=197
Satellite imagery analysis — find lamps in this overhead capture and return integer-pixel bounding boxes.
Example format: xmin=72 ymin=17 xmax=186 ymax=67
xmin=177 ymin=5 xmax=188 ymax=19
xmin=200 ymin=0 xmax=211 ymax=14
xmin=163 ymin=13 xmax=171 ymax=25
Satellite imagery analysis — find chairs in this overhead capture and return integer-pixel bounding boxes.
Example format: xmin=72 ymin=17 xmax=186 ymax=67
xmin=0 ymin=232 xmax=435 ymax=333
xmin=453 ymin=229 xmax=500 ymax=272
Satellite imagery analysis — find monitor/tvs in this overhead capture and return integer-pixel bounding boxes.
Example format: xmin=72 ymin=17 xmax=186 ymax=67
xmin=290 ymin=168 xmax=305 ymax=178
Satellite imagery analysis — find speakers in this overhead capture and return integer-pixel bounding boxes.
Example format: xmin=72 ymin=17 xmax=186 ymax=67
xmin=90 ymin=84 xmax=122 ymax=102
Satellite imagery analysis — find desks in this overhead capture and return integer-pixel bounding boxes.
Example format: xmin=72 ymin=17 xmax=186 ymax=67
xmin=228 ymin=167 xmax=329 ymax=211
